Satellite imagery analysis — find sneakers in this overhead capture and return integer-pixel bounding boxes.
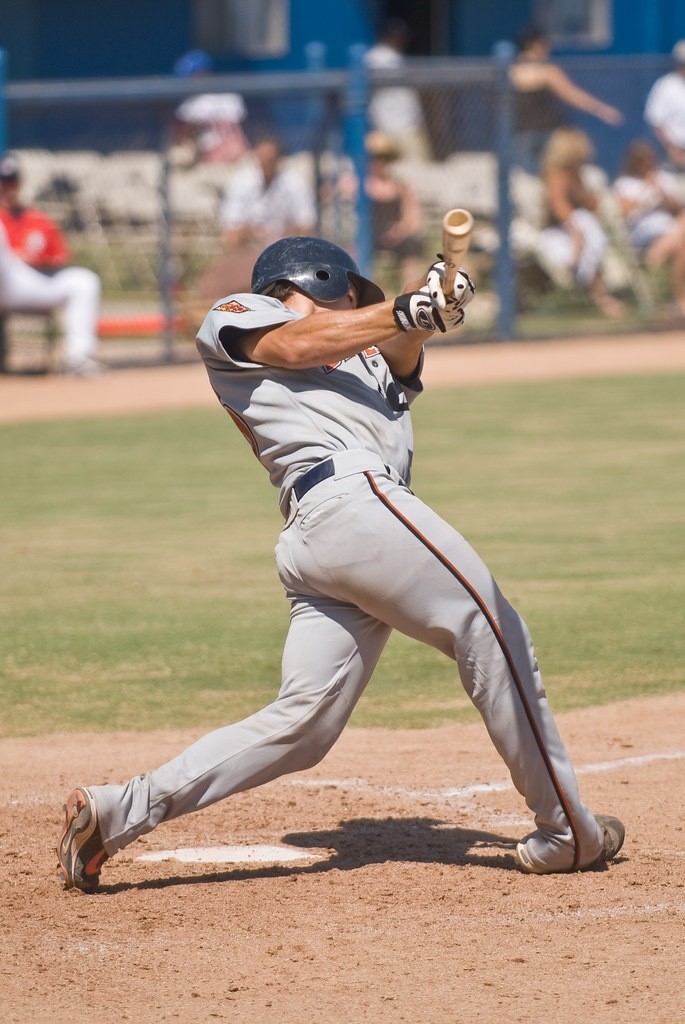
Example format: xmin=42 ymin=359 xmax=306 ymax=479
xmin=516 ymin=814 xmax=626 ymax=873
xmin=56 ymin=787 xmax=110 ymax=893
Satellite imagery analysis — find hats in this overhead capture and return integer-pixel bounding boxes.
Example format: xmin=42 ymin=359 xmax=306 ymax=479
xmin=0 ymin=154 xmax=19 ymax=177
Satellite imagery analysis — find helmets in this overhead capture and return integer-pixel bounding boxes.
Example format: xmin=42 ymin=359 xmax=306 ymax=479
xmin=251 ymin=236 xmax=385 ymax=308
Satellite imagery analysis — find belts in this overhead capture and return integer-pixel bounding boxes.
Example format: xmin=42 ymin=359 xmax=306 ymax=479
xmin=287 ymin=459 xmax=390 ymax=512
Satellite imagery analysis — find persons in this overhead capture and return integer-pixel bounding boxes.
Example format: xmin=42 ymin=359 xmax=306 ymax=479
xmin=169 ymin=24 xmax=685 ymax=332
xmin=0 ymin=154 xmax=106 ymax=379
xmin=56 ymin=238 xmax=626 ymax=893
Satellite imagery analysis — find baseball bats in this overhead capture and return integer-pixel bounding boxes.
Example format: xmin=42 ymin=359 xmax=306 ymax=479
xmin=442 ymin=209 xmax=474 ymax=295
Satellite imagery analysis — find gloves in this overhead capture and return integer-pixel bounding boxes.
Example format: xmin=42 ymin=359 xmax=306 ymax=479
xmin=392 ymin=285 xmax=464 ymax=333
xmin=426 ymin=254 xmax=476 ymax=309
